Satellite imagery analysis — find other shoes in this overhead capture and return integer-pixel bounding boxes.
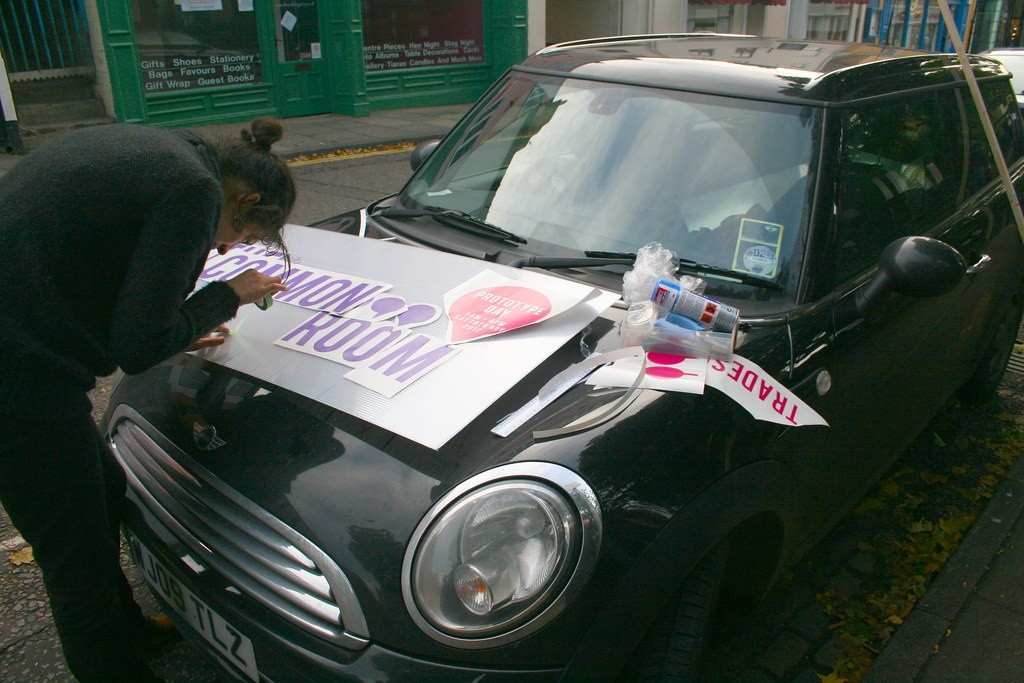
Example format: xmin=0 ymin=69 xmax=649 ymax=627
xmin=133 ymin=613 xmax=174 ymax=645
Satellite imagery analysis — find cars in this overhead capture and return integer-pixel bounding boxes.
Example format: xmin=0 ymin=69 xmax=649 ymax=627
xmin=85 ymin=31 xmax=1024 ymax=683
xmin=977 ymin=45 xmax=1024 ymax=115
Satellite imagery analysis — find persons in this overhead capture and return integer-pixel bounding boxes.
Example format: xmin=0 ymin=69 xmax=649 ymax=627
xmin=0 ymin=119 xmax=296 ymax=683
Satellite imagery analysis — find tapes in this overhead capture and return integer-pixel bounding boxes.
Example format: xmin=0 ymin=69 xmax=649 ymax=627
xmin=253 ymin=293 xmax=275 ymax=311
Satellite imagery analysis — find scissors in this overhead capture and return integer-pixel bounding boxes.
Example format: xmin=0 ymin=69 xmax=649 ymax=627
xmin=654 ymin=311 xmax=733 ymax=351
